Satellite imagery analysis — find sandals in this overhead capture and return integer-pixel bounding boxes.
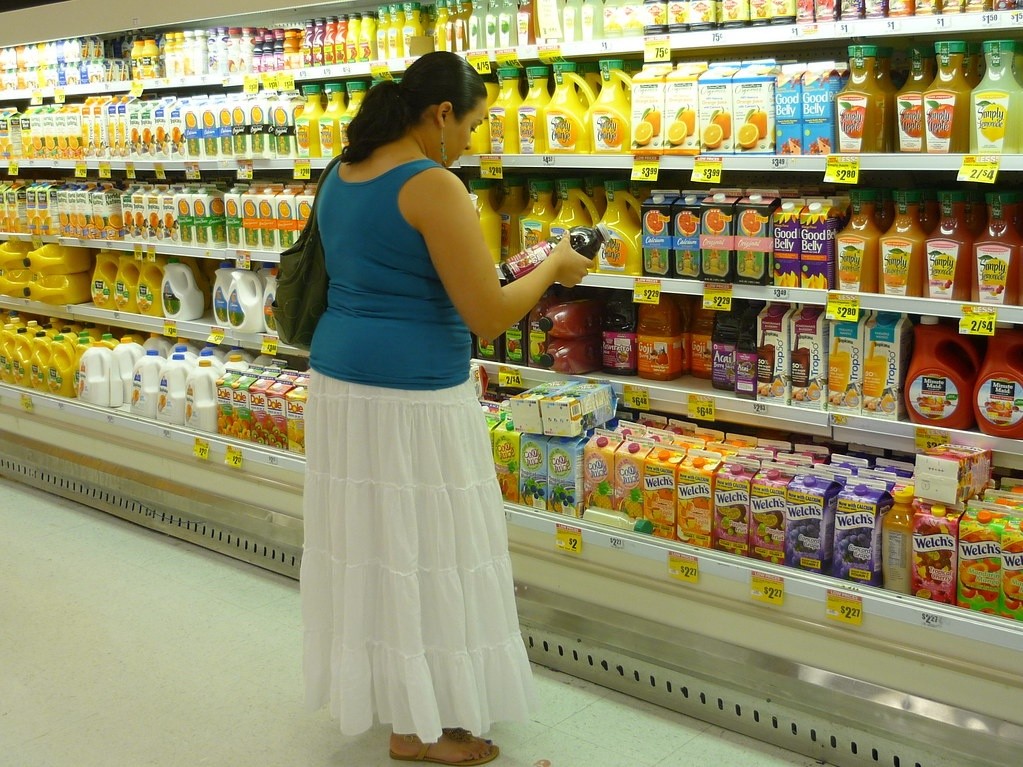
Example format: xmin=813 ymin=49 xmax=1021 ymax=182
xmin=390 ymin=728 xmax=499 ymax=766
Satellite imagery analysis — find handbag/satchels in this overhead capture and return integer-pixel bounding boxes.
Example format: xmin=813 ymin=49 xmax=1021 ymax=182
xmin=273 ymin=153 xmax=347 ymax=348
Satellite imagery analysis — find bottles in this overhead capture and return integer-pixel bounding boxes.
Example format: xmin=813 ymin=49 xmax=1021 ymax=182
xmin=0 ymin=240 xmax=278 ymax=432
xmin=962 ymin=42 xmax=980 ymax=87
xmin=458 ymin=81 xmax=497 ymax=153
xmin=970 ymin=40 xmax=1023 ymax=154
xmin=896 ymin=46 xmax=932 ymax=153
xmin=467 ymin=175 xmax=653 ymax=283
xmin=904 ymin=315 xmax=1023 ymax=440
xmin=577 ymin=60 xmax=602 ymax=108
xmin=583 ymin=506 xmax=655 ymax=534
xmin=834 ymin=45 xmax=879 ymax=154
xmin=295 ymin=81 xmax=367 ymax=159
xmin=518 ymin=66 xmax=551 ymax=154
xmin=587 ymin=60 xmax=631 ymax=155
xmin=372 ymin=81 xmax=386 ymax=90
xmin=623 ymin=60 xmax=642 ymax=104
xmin=922 ymin=41 xmax=973 ymax=153
xmin=835 ymin=185 xmax=1023 ymax=306
xmin=474 ymin=296 xmax=764 ymax=400
xmin=1011 ymin=41 xmax=1023 ymax=89
xmin=542 ymin=62 xmax=596 ymax=154
xmin=389 ymin=76 xmax=401 ymax=84
xmin=132 ymin=0 xmax=1023 ymax=80
xmin=488 ymin=67 xmax=523 ymax=154
xmin=869 ymin=46 xmax=895 ymax=153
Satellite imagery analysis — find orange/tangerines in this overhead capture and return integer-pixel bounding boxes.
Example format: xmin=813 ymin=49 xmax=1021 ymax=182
xmin=522 ymin=228 xmax=625 ymax=268
xmin=297 ymin=123 xmax=349 ymax=148
xmin=489 ymin=105 xmax=767 ymax=149
xmin=0 ymin=121 xmax=179 ymax=239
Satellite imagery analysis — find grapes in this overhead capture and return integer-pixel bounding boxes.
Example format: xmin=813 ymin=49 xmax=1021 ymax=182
xmin=788 ymin=517 xmax=872 ymax=585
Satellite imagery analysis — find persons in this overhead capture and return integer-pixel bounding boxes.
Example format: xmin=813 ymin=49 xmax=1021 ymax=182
xmin=294 ymin=51 xmax=594 ymax=765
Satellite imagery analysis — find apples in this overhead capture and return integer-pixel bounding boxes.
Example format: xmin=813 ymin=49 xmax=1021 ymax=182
xmin=839 ymin=103 xmax=953 ymax=140
